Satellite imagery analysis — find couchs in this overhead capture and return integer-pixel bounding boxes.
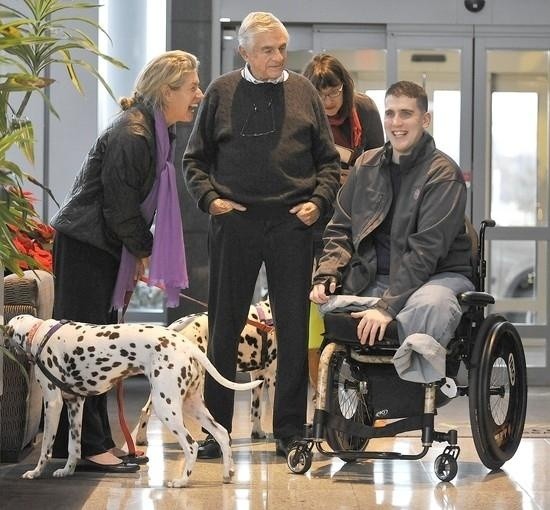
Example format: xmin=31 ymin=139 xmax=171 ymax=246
xmin=0 ymin=266 xmax=57 ymax=465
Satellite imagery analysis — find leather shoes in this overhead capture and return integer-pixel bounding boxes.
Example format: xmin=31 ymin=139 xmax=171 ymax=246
xmin=79 ymin=459 xmax=140 ymax=471
xmin=197 ymin=433 xmax=233 ymax=459
xmin=118 ymin=454 xmax=149 ymax=463
xmin=276 ymin=439 xmax=313 ymax=461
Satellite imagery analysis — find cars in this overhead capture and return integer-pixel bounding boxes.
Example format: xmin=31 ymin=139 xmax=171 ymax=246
xmin=498 ymin=266 xmax=535 ymax=325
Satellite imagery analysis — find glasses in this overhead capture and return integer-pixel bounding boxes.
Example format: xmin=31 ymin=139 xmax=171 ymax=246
xmin=317 ymin=82 xmax=343 ymax=101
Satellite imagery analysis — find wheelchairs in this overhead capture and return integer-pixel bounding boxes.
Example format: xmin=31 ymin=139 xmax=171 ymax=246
xmin=284 ymin=215 xmax=527 ymax=485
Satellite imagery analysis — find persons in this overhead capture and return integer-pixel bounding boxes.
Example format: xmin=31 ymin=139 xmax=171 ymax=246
xmin=181 ymin=13 xmax=341 ymax=460
xmin=302 ymin=55 xmax=384 ymax=402
xmin=49 ymin=49 xmax=205 ymax=473
xmin=309 ymin=80 xmax=474 ymax=385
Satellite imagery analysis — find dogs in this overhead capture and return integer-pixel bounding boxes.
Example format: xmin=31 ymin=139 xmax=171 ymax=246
xmin=135 ymin=292 xmax=278 ymax=445
xmin=0 ymin=314 xmax=265 ymax=488
